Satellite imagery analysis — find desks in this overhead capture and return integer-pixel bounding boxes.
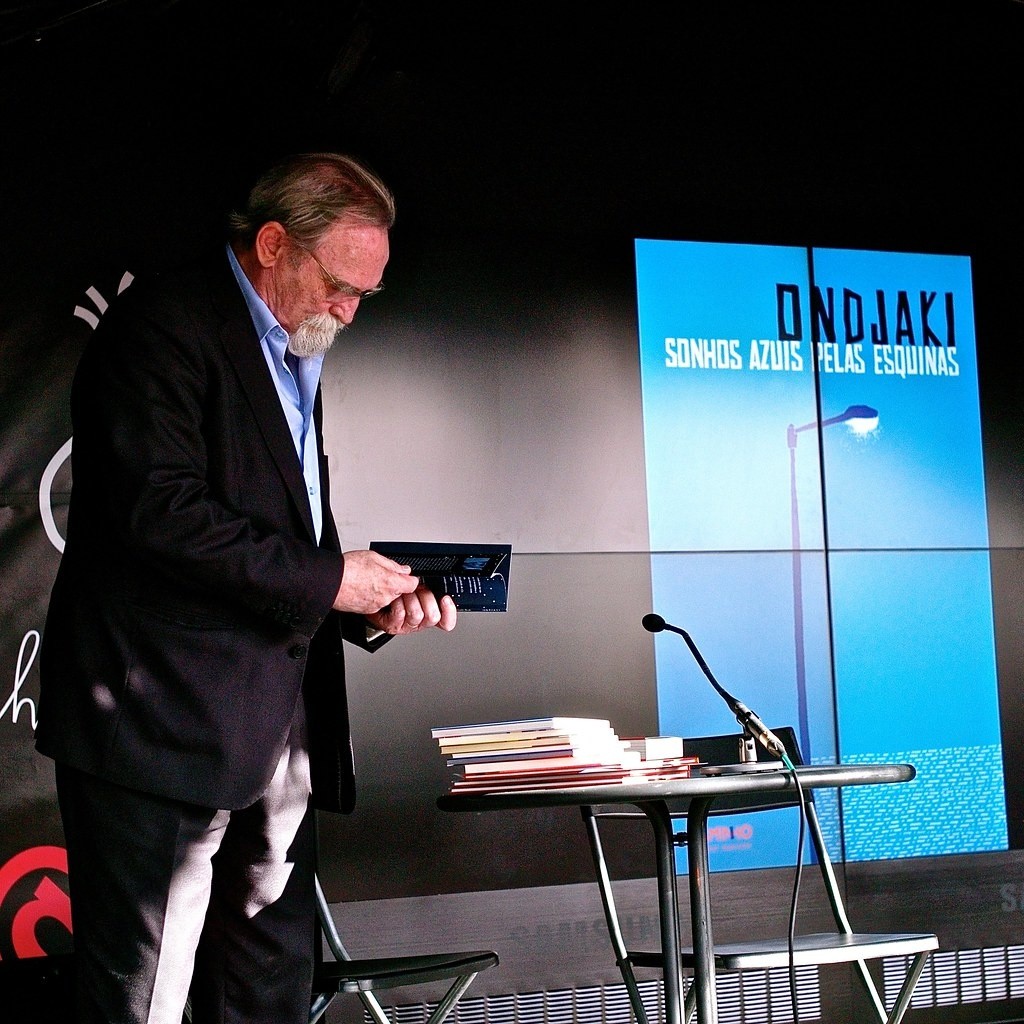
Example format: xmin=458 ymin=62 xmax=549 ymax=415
xmin=437 ymin=764 xmax=916 ymax=1024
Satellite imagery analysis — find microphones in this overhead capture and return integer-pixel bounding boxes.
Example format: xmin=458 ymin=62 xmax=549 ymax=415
xmin=642 ymin=614 xmax=786 ymax=757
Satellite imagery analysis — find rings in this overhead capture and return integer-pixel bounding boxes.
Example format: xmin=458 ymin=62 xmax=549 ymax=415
xmin=405 ymin=622 xmax=419 ymax=629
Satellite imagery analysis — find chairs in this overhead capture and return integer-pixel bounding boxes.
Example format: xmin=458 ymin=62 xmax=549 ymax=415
xmin=581 ymin=727 xmax=940 ymax=1024
xmin=308 ymin=870 xmax=498 ymax=1024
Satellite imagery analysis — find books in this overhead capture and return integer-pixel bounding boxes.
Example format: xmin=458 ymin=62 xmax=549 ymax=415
xmin=429 ymin=715 xmax=703 ymax=799
xmin=368 ymin=540 xmax=512 ymax=613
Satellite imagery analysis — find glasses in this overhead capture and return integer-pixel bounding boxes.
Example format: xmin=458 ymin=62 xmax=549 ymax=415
xmin=279 ymin=223 xmax=385 ymax=298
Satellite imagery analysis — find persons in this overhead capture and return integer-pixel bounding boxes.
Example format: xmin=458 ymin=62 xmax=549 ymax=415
xmin=31 ymin=150 xmax=457 ymax=1024
